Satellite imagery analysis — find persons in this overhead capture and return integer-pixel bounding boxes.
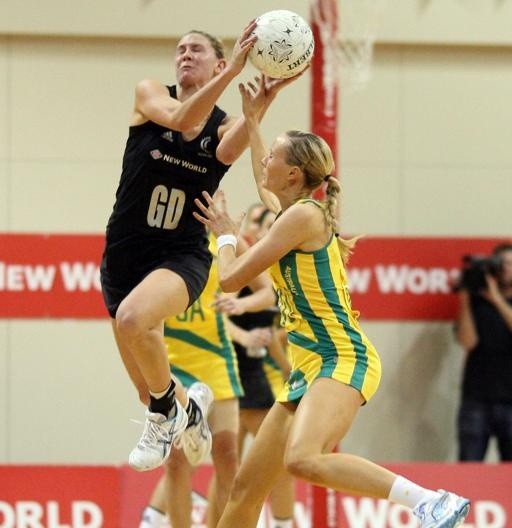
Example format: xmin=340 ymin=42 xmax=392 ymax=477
xmin=99 ymin=19 xmax=315 ymax=472
xmin=139 ymin=191 xmax=299 ymax=527
xmin=190 ymin=72 xmax=471 ymax=527
xmin=451 ymin=242 xmax=512 ymax=463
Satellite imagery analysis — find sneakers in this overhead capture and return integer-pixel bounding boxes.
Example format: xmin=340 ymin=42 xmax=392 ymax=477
xmin=179 ymin=381 xmax=214 ymax=466
xmin=127 ymin=397 xmax=189 ymax=471
xmin=416 ymin=488 xmax=471 ymax=528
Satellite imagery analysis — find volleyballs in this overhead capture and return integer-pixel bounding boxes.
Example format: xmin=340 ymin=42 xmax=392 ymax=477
xmin=245 ymin=9 xmax=318 ymax=80
